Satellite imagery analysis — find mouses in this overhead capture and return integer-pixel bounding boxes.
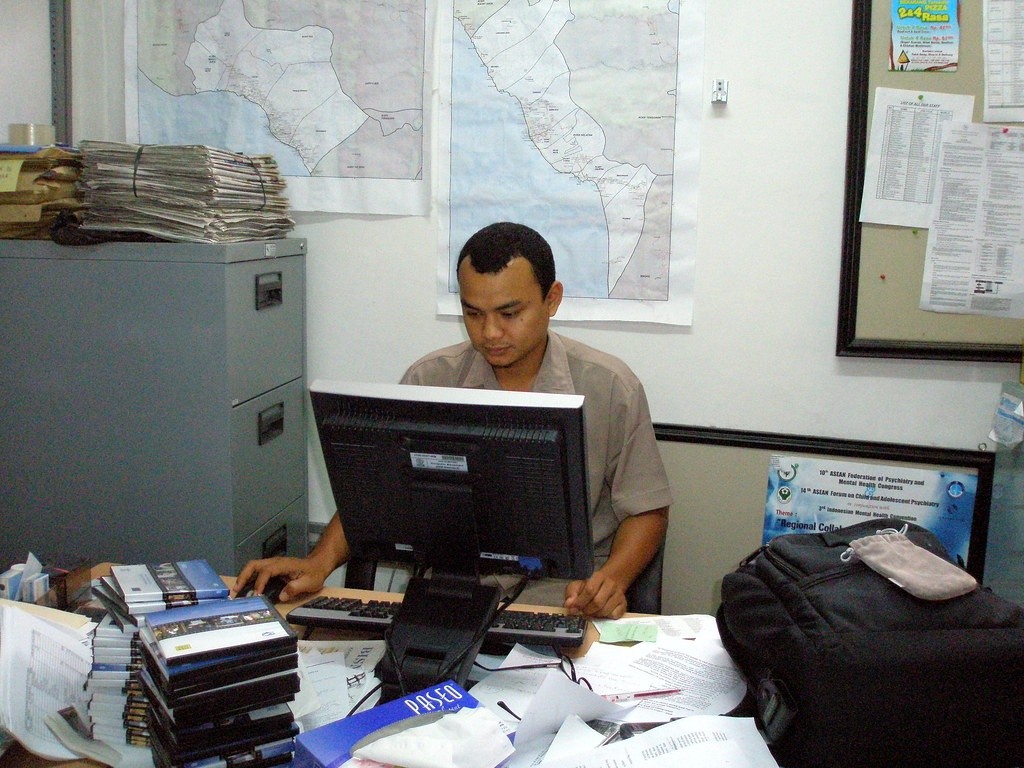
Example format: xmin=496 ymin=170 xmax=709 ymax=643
xmin=235 ymin=577 xmax=290 ymax=605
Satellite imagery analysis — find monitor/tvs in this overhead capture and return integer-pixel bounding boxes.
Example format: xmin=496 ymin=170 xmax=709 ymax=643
xmin=309 ymin=381 xmax=595 ymax=710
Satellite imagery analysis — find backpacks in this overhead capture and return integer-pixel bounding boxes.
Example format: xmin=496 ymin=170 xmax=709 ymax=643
xmin=716 ymin=517 xmax=1022 ymax=767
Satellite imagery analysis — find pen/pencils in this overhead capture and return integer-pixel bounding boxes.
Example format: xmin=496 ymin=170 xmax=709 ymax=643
xmin=600 ymin=689 xmax=682 ymax=703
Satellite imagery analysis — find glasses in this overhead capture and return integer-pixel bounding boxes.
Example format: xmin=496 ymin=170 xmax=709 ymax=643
xmin=474 ymin=655 xmax=593 ymax=720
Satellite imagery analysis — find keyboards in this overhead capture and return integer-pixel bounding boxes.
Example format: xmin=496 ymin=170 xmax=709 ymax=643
xmin=285 ymin=597 xmax=588 ymax=648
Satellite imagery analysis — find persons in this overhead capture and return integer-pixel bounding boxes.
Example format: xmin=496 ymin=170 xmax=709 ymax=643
xmin=233 ymin=222 xmax=666 ymax=623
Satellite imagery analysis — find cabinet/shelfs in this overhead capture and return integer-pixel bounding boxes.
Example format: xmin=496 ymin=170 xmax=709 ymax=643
xmin=0 ymin=239 xmax=309 ymax=577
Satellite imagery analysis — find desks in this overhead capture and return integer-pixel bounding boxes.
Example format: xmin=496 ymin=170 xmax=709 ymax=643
xmin=0 ymin=562 xmax=663 ymax=768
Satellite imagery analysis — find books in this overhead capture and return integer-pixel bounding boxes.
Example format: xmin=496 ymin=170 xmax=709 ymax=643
xmin=0 ymin=142 xmax=295 ymax=245
xmin=0 ymin=553 xmax=777 ymax=768
